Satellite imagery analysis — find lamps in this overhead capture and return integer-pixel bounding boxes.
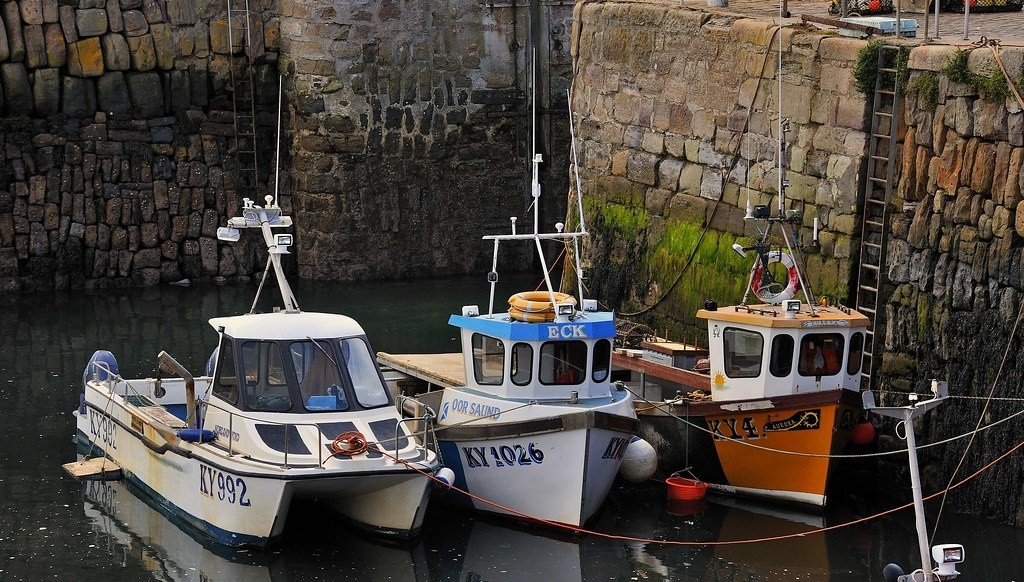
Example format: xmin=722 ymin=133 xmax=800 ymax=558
xmin=554 ymin=304 xmax=573 ymax=324
xmin=582 ymin=299 xmax=597 ymax=312
xmin=273 ymin=231 xmax=293 ymax=254
xmin=462 ymin=304 xmax=479 ymax=318
xmin=732 ymin=243 xmax=754 ymax=257
xmin=782 ymin=299 xmax=802 ymax=318
xmin=932 ymin=542 xmax=965 ymax=575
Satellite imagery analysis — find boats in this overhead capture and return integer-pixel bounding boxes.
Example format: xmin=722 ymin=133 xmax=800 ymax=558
xmin=604 ymin=0 xmax=878 ymax=517
xmin=70 ymin=75 xmax=445 ymax=562
xmin=374 ymin=45 xmax=638 ymax=540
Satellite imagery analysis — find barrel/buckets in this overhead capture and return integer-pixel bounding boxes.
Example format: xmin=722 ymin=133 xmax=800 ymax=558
xmin=665 ymin=468 xmax=709 ymax=501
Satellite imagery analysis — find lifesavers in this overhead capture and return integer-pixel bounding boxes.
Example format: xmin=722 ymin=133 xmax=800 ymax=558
xmin=750 ymin=250 xmax=799 ymax=305
xmin=508 ymin=306 xmax=577 ymax=322
xmin=509 ymin=290 xmax=577 ymax=313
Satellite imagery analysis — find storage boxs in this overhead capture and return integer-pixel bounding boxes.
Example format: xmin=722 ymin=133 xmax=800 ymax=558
xmin=840 ymin=16 xmax=917 ymax=38
